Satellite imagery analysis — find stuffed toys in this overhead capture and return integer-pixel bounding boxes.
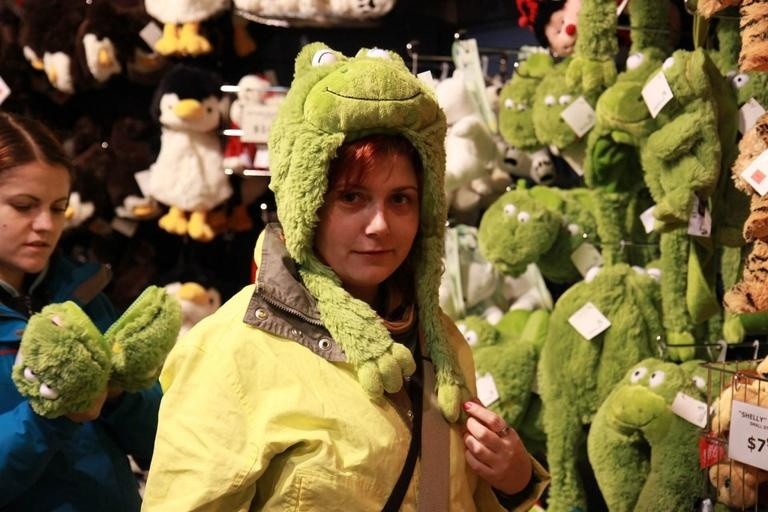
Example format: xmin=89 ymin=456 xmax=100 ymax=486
xmin=25 ymin=0 xmax=767 ymax=512
xmin=0 ymin=0 xmax=768 ymax=512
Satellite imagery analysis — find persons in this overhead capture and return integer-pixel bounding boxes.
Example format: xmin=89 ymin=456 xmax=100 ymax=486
xmin=2 ymin=0 xmax=553 ymax=511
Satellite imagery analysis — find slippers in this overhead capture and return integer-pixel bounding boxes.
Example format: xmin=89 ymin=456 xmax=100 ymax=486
xmin=499 ymin=53 xmax=594 ymax=149
xmin=454 ymin=307 xmax=550 ymax=427
xmin=103 ymin=285 xmax=183 ymax=393
xmin=477 ymin=184 xmax=600 ymax=281
xmin=12 ymin=301 xmax=112 ymax=421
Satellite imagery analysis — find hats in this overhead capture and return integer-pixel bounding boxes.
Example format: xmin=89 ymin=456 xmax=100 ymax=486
xmin=267 ymin=42 xmax=472 ymax=423
